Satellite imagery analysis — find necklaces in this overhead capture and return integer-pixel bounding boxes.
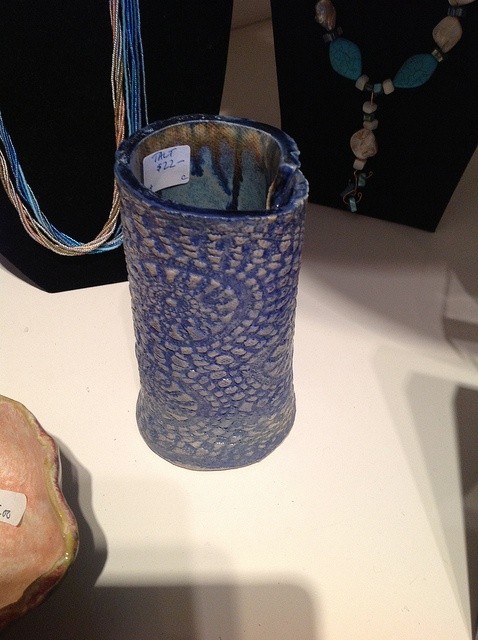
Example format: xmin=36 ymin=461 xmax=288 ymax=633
xmin=313 ymin=2 xmax=468 ymax=212
xmin=1 ymin=1 xmax=156 ymax=260
